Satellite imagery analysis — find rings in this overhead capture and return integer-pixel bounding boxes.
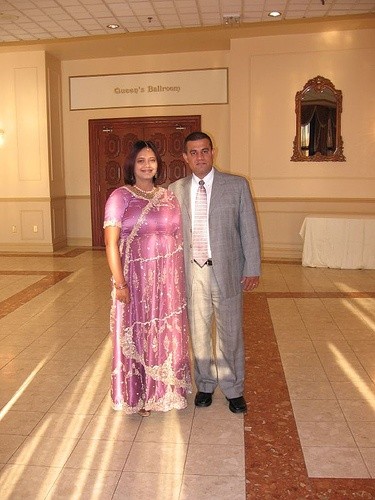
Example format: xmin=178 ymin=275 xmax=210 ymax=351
xmin=252 ymin=283 xmax=256 ymax=286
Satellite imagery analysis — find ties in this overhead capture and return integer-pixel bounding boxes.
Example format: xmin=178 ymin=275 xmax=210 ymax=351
xmin=193 ymin=180 xmax=209 ymax=269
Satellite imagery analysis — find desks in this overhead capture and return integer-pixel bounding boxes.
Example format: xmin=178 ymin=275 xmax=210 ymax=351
xmin=299 ymin=214 xmax=375 ymax=270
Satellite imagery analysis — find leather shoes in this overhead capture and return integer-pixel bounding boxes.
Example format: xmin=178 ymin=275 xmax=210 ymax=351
xmin=193 ymin=389 xmax=215 ymax=409
xmin=224 ymin=395 xmax=247 ymax=413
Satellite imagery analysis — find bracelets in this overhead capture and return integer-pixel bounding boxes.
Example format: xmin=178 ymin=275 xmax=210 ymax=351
xmin=114 ymin=281 xmax=127 ymax=290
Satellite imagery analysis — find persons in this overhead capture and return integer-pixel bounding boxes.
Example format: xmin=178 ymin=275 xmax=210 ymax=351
xmin=103 ymin=139 xmax=193 ymax=417
xmin=167 ymin=131 xmax=262 ymax=414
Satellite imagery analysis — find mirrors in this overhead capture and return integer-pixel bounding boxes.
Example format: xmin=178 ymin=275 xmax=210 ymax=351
xmin=290 ymin=75 xmax=347 ymax=163
